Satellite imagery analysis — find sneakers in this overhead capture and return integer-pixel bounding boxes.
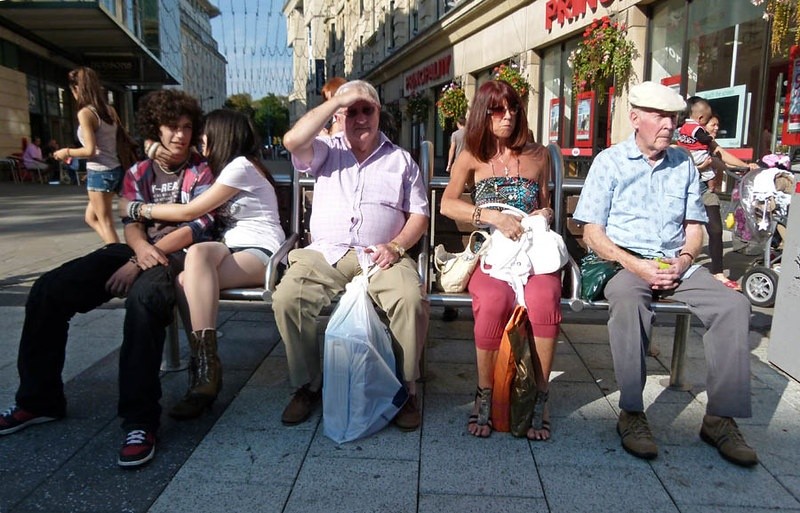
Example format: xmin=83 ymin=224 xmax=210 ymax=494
xmin=281 ymin=383 xmax=323 ymax=425
xmin=615 ymin=410 xmax=658 ymax=459
xmin=698 ymin=411 xmax=758 ymax=468
xmin=0 ymin=402 xmax=67 ymax=434
xmin=118 ymin=413 xmax=155 ymax=466
xmin=396 ymin=394 xmax=420 ymax=431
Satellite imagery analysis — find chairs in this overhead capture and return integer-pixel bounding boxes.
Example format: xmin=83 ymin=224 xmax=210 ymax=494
xmin=7 ymin=153 xmax=86 ymax=186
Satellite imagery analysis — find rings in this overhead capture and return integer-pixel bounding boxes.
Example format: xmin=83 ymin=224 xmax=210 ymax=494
xmin=513 ymin=229 xmax=524 ymax=240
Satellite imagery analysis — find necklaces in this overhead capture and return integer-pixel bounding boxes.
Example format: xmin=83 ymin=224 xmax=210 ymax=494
xmin=486 ymin=148 xmax=521 ymax=201
xmin=495 ymin=149 xmax=513 ymax=177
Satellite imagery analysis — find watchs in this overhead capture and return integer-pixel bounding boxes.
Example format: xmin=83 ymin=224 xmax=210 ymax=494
xmin=679 ymin=252 xmax=696 ymax=266
xmin=390 ymin=240 xmax=407 ymax=258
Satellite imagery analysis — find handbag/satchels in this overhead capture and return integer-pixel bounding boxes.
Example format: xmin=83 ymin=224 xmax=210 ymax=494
xmin=564 ymin=244 xmax=642 ymax=302
xmin=433 ymin=229 xmax=489 ymax=293
xmin=322 ymin=246 xmax=403 ymax=444
xmin=472 ymin=202 xmax=569 ymax=280
xmin=116 ymin=127 xmax=139 ymax=171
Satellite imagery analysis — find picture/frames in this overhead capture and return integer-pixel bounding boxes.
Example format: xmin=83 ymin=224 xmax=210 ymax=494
xmin=783 ymin=45 xmax=800 ymax=147
xmin=661 ymin=76 xmax=681 ymax=96
xmin=573 ymin=91 xmax=595 ymax=148
xmin=549 ymin=98 xmax=560 ymax=144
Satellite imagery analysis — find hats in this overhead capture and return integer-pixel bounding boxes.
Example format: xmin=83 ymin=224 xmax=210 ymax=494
xmin=630 ymin=81 xmax=687 ymax=112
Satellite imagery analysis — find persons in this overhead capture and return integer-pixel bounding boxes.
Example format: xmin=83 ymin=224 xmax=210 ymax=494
xmin=438 ymin=79 xmax=575 ymax=444
xmin=445 ymin=114 xmax=470 ymax=173
xmin=52 ymin=69 xmax=128 ymax=245
xmin=671 ymin=96 xmax=761 ymax=292
xmin=0 ymin=87 xmax=219 ymax=469
xmin=116 ymin=106 xmax=291 ymax=423
xmin=701 ymin=116 xmax=720 ymax=140
xmin=22 ymin=134 xmax=65 ymax=185
xmin=678 ymin=101 xmax=723 ymax=198
xmin=571 ymin=81 xmax=762 ymax=470
xmin=321 ymin=78 xmax=352 ymax=137
xmin=273 ymin=79 xmax=429 ymax=430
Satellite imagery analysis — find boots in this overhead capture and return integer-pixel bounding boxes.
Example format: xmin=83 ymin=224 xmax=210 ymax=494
xmin=169 ymin=382 xmax=222 ymax=418
xmin=188 ymin=328 xmax=223 ymax=398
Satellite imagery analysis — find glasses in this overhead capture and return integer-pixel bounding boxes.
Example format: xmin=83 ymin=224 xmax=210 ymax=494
xmin=339 ymin=106 xmax=377 ymax=116
xmin=486 ymin=104 xmax=519 ymax=116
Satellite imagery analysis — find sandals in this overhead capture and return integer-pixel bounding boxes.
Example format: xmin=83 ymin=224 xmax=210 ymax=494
xmin=468 ymin=385 xmax=493 ymax=438
xmin=526 ymin=390 xmax=551 ymax=440
xmin=722 ymin=280 xmax=742 ymax=290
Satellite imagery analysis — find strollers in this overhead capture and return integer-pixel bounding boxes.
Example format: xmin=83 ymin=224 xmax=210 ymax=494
xmin=739 ymin=165 xmax=797 ymax=308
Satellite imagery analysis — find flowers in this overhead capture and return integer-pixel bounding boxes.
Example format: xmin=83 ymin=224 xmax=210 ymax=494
xmin=566 ymin=14 xmax=635 ymax=105
xmin=404 ymin=90 xmax=433 ymax=123
xmin=751 ymin=0 xmax=800 ymax=58
xmin=493 ymin=61 xmax=542 ymax=99
xmin=436 ymin=82 xmax=468 ymax=132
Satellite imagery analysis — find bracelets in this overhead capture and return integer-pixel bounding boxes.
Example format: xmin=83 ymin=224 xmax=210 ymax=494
xmin=130 ymin=256 xmax=142 ymax=268
xmin=469 ymin=204 xmax=483 ymax=227
xmin=148 ymin=142 xmax=160 ymax=160
xmin=66 ymin=147 xmax=71 ymax=157
xmin=126 ymin=201 xmax=156 ymax=221
xmin=544 ymin=207 xmax=552 ymax=226
xmin=143 ymin=140 xmax=154 ymax=155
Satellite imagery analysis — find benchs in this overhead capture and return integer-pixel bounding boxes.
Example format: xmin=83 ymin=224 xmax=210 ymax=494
xmin=156 ymin=141 xmax=752 ymax=391
